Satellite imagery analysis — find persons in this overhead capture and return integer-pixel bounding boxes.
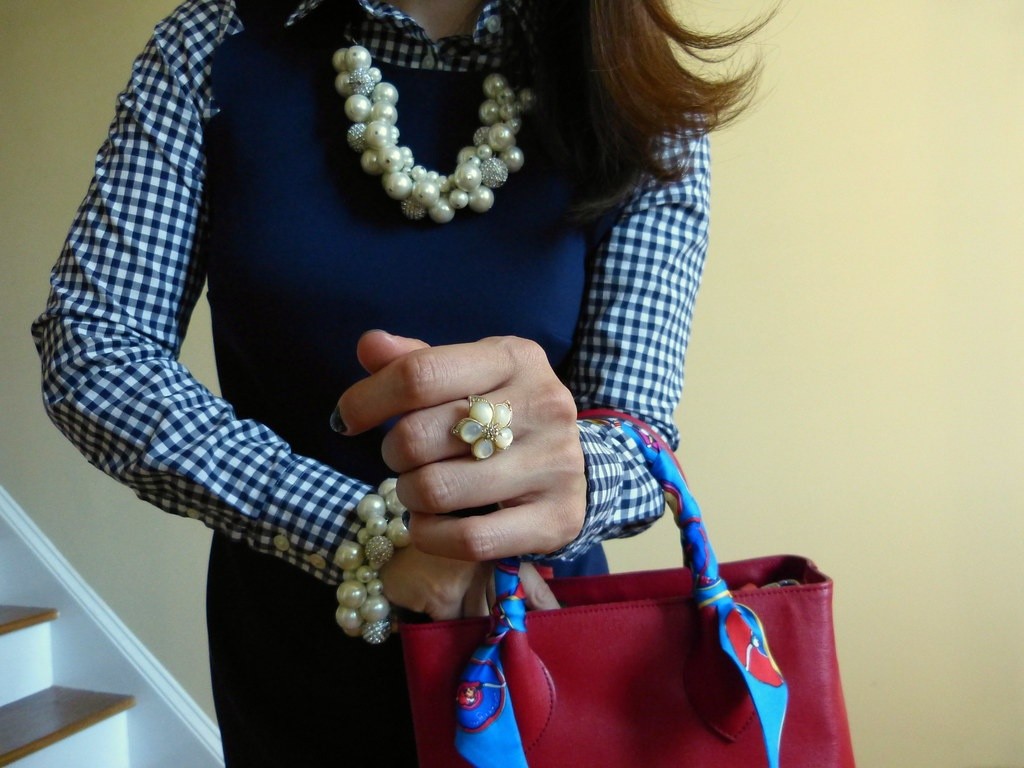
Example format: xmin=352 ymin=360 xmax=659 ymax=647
xmin=30 ymin=0 xmax=773 ymax=767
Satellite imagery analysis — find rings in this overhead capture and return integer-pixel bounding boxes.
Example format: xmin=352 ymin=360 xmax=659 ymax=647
xmin=450 ymin=395 xmax=515 ymax=463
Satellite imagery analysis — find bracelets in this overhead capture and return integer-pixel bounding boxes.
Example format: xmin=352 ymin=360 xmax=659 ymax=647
xmin=336 ymin=478 xmax=413 ymax=644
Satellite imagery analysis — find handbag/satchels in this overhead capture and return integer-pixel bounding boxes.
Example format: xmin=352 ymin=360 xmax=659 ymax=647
xmin=399 ymin=409 xmax=855 ymax=768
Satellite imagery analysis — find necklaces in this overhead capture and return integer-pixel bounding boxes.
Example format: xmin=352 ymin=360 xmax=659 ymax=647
xmin=332 ymin=24 xmax=534 ymax=223
